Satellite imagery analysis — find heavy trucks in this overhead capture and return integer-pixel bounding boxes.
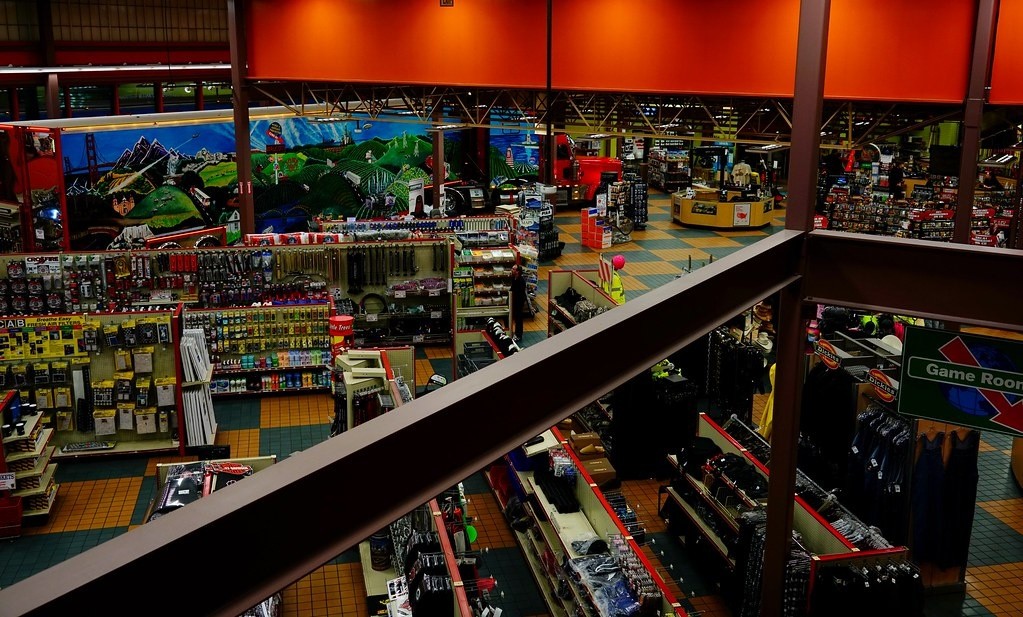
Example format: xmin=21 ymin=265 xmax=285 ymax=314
xmin=0 ymin=93 xmax=625 ymax=255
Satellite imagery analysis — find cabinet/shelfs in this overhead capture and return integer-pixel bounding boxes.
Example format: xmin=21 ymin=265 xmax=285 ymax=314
xmin=667 ymin=413 xmax=908 ymax=617
xmin=547 ymin=269 xmax=620 ymax=337
xmin=0 ymin=390 xmax=61 ymax=527
xmin=648 ymin=148 xmax=692 ymax=193
xmin=209 ymin=364 xmax=334 ymax=397
xmin=814 ymin=147 xmax=1023 ymax=250
xmin=454 ymin=242 xmax=520 ymax=307
xmin=350 ymin=312 xmax=453 ymax=345
xmin=452 ymin=330 xmax=688 ymax=617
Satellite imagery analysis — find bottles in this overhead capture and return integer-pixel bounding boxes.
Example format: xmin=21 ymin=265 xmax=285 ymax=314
xmin=286 ymin=371 xmax=322 ymax=387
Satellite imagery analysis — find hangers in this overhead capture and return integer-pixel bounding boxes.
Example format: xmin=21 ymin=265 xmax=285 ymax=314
xmin=916 ymin=421 xmax=948 ymax=444
xmin=724 ymin=421 xmax=896 ymax=550
xmin=857 ymin=400 xmax=914 ymax=445
xmin=947 ymin=426 xmax=981 ymax=441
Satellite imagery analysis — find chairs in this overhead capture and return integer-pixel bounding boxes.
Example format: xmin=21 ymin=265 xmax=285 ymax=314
xmin=416 ymin=374 xmax=448 ymax=399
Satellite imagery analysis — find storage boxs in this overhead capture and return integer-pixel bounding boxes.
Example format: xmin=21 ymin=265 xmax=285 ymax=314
xmin=568 ymin=432 xmax=615 ymax=483
xmin=457 ymin=341 xmax=497 ymax=377
xmin=581 ymin=207 xmax=612 ymax=248
